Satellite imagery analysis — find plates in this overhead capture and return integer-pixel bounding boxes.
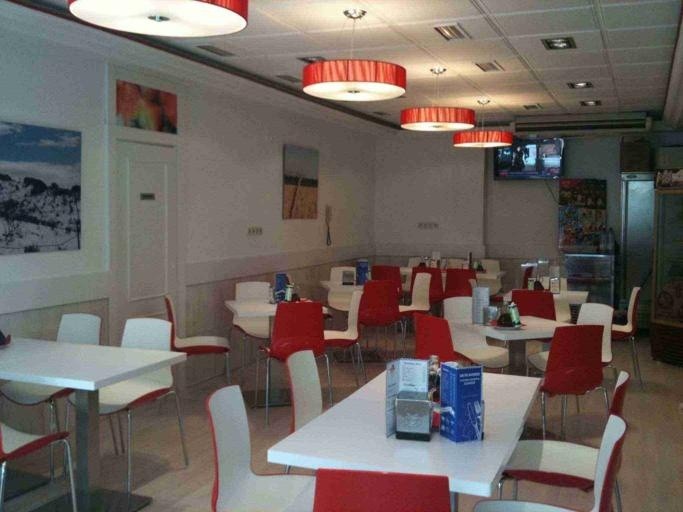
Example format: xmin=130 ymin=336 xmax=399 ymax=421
xmin=492 ymin=322 xmax=526 ymax=329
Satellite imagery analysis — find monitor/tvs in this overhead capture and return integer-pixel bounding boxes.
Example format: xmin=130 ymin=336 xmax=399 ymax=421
xmin=493 ymin=137 xmax=566 ymax=179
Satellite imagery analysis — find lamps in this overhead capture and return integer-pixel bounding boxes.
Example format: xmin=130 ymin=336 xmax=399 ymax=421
xmin=400 ymin=61 xmax=515 ymax=150
xmin=64 ymin=0 xmax=249 ymax=42
xmin=300 ymin=5 xmax=411 ymax=104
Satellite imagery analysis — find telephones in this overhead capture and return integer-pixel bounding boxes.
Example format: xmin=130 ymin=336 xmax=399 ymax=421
xmin=326 ymin=207 xmax=332 ymax=225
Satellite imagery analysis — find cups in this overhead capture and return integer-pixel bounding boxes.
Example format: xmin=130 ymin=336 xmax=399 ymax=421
xmin=483 ymin=306 xmax=497 ymax=326
xmin=342 ymin=270 xmax=354 ymax=285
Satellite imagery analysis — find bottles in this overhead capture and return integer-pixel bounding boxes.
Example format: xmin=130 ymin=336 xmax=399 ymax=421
xmin=285 ymin=285 xmax=293 ymax=301
xmin=427 ymin=354 xmax=440 ymax=401
xmin=509 ymin=303 xmax=522 ymax=329
xmin=528 ymin=278 xmax=533 ymax=290
xmin=564 ymin=227 xmax=615 ymax=254
xmin=418 ymin=256 xmax=446 ymax=272
